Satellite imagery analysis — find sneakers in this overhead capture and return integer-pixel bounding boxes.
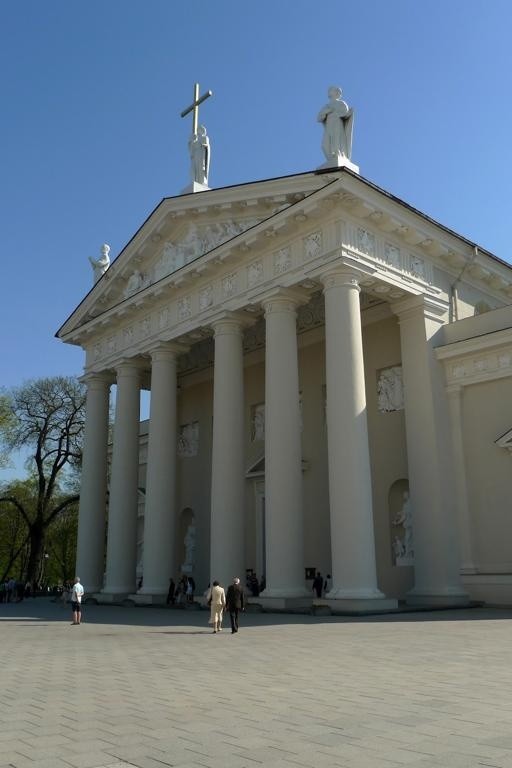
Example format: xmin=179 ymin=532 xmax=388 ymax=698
xmin=218 ymin=628 xmax=222 ymax=631
xmin=213 ymin=631 xmax=216 ymax=633
xmin=71 ymin=622 xmax=80 ymax=625
xmin=232 ymin=629 xmax=238 ymax=634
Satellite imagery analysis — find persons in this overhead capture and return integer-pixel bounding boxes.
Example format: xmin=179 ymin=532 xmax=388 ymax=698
xmin=323 ymin=574 xmax=332 ymax=593
xmin=312 ymin=572 xmax=323 ymax=597
xmin=88 ymin=243 xmax=111 ymax=284
xmin=0 ymin=577 xmax=16 ymax=603
xmin=391 ymin=490 xmax=412 ymax=558
xmin=167 ymin=568 xmax=266 ymax=607
xmin=61 ymin=578 xmax=76 ymax=608
xmin=206 ymin=580 xmax=225 ymax=633
xmin=393 ymin=534 xmax=405 ymax=557
xmin=315 ymin=84 xmax=354 ymax=159
xmin=138 ymin=576 xmax=143 ymax=588
xmin=188 ymin=125 xmax=211 ymax=186
xmin=70 ymin=576 xmax=84 ymax=626
xmin=225 ymin=577 xmax=244 ymax=634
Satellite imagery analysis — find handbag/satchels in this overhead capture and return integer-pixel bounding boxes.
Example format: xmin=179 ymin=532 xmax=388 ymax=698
xmin=207 ymin=588 xmax=213 ymax=606
